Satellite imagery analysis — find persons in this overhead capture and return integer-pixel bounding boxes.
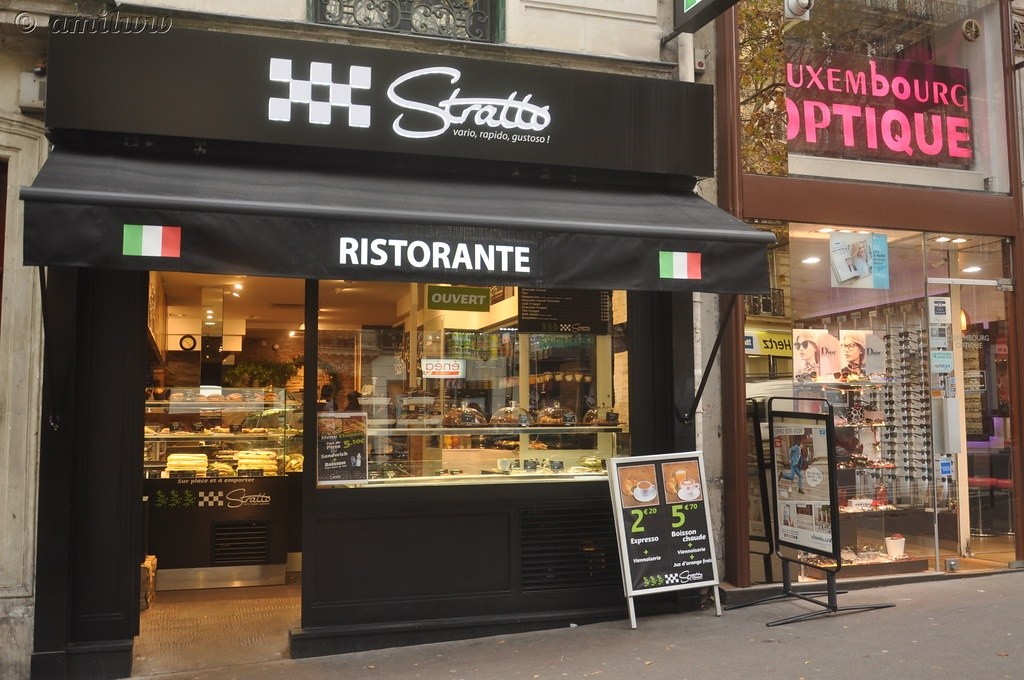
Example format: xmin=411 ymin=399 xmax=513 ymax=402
xmin=320 ymin=384 xmax=338 ymax=411
xmin=469 ymin=403 xmax=492 ymax=424
xmin=344 ymin=391 xmax=361 ymax=410
xmin=797 ymin=332 xmax=820 ymax=380
xmin=841 ymin=332 xmax=865 ymax=378
xmin=778 ymin=441 xmax=805 ymax=495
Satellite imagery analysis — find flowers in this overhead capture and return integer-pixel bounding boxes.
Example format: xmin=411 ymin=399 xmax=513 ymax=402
xmin=891 ymin=533 xmax=902 ymax=540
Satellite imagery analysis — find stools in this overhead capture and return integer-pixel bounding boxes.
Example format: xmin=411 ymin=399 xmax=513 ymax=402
xmin=968 ymin=477 xmax=1014 ymax=537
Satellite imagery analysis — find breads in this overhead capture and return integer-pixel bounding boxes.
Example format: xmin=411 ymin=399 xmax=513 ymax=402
xmin=665 ymin=477 xmax=677 ymax=494
xmin=621 ymin=479 xmax=639 ymax=496
xmin=144 ymin=387 xmax=303 ymax=477
xmin=441 ymin=410 xmax=614 ymax=425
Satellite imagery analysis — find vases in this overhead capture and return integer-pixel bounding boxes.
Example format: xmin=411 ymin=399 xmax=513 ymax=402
xmin=884 ymin=537 xmax=906 ymax=560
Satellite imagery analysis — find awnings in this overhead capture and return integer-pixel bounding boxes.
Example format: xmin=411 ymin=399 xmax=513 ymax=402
xmin=20 ymin=153 xmax=775 ymax=295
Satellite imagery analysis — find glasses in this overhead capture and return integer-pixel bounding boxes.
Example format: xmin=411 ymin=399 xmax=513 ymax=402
xmin=936 ymin=347 xmax=947 ymax=351
xmin=841 ymin=343 xmax=854 ymax=352
xmin=939 ymin=373 xmax=948 ymax=376
xmin=796 ymin=372 xmax=816 ymax=383
xmin=856 ymin=330 xmax=933 ymax=482
xmin=825 ymin=372 xmax=848 ymax=379
xmin=794 ymin=341 xmax=808 ymax=350
xmin=935 ymin=453 xmax=955 ymax=483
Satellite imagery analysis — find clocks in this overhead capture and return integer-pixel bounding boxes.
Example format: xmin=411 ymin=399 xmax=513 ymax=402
xmin=180 ymin=335 xmax=196 ymax=351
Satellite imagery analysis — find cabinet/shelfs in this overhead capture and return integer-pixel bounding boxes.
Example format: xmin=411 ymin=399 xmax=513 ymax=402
xmin=795 ymin=382 xmax=930 ymax=580
xmin=320 ymin=346 xmax=622 ymax=474
xmin=141 ymin=386 xmax=305 ymax=478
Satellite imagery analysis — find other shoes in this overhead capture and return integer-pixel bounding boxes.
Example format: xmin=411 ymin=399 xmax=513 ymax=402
xmin=798 ymin=489 xmax=804 ymax=494
xmin=778 ymin=472 xmax=783 ymax=481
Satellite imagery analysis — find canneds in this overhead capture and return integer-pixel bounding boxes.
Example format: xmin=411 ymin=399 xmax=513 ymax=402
xmin=444 ymin=332 xmax=499 ymax=360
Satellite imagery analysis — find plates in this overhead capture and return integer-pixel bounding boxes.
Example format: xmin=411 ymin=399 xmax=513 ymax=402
xmin=678 ymin=489 xmax=700 ymax=501
xmin=634 ymin=486 xmax=657 ymax=502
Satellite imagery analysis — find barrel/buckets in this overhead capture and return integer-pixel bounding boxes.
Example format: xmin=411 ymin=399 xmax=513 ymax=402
xmin=885 ymin=537 xmax=906 ymax=558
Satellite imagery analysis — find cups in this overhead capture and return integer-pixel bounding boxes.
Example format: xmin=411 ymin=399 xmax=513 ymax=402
xmin=676 ymin=470 xmax=687 ymax=488
xmin=637 ymin=481 xmax=655 ymax=496
xmin=680 ymin=478 xmax=699 ymax=495
xmin=496 ymin=459 xmax=510 ymax=470
xmin=989 ymin=436 xmax=1004 ymax=449
